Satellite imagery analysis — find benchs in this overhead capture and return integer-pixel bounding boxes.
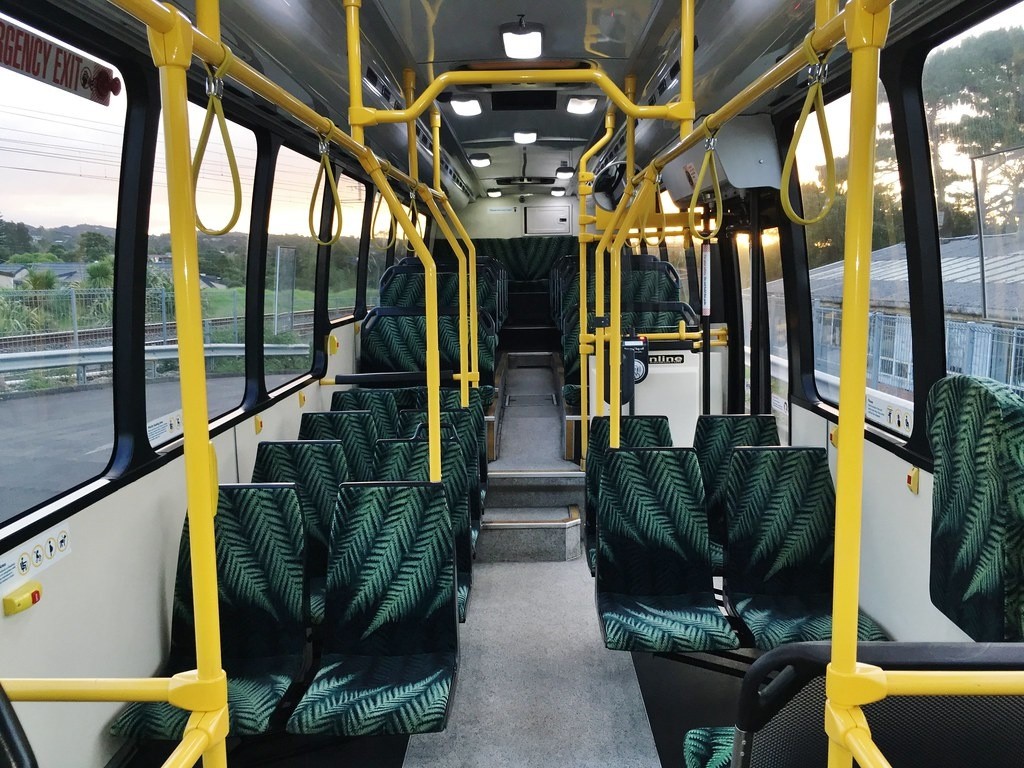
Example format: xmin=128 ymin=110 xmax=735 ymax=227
xmin=560 ymin=261 xmax=679 ymax=347
xmin=682 ymin=641 xmax=1024 ymax=768
xmin=378 ymin=264 xmax=499 ymax=348
xmin=399 ymin=256 xmax=503 ymax=329
xmin=561 ymin=300 xmax=701 ymax=417
xmin=556 ymin=255 xmax=660 ymax=332
xmin=360 ymin=305 xmax=498 ymax=407
xmin=425 ymin=234 xmax=626 ymax=292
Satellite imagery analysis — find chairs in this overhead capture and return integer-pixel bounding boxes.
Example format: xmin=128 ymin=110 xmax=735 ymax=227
xmin=249 ymin=440 xmax=351 ymax=630
xmin=415 ymin=387 xmax=489 ymax=516
xmin=284 ymin=482 xmax=461 ymax=739
xmin=109 ymin=481 xmax=308 ymax=741
xmin=395 ymin=408 xmax=483 ymax=561
xmin=584 ymin=416 xmax=673 ymax=578
xmin=722 ymin=445 xmax=892 ymax=653
xmin=329 ymin=390 xmax=403 ymax=439
xmin=693 ymin=414 xmax=782 ymax=577
xmin=594 ymin=446 xmax=740 ymax=652
xmin=298 ymin=410 xmax=379 ymax=482
xmin=368 ymin=438 xmax=473 ymax=624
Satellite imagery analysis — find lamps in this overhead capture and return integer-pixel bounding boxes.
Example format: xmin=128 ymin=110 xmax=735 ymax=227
xmin=487 ymin=188 xmax=502 ymax=198
xmin=498 ymin=22 xmax=544 ymax=60
xmin=556 ymin=167 xmax=575 ymax=179
xmin=469 ymin=153 xmax=491 ymax=168
xmin=512 ymin=128 xmax=538 ymax=145
xmin=565 ymin=95 xmax=597 ymax=115
xmin=449 ymin=94 xmax=483 ymax=116
xmin=550 ymin=187 xmax=566 ymax=197
